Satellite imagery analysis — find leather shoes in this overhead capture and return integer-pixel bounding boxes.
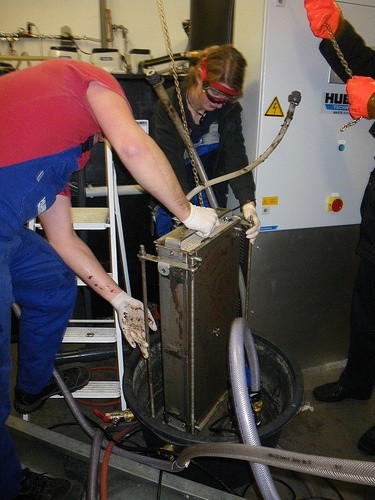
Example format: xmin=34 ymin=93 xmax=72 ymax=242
xmin=358 ymin=424 xmax=375 ymax=455
xmin=313 ymin=380 xmax=372 ymax=403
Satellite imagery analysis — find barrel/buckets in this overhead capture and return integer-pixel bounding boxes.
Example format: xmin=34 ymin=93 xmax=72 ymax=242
xmin=122 ymin=324 xmax=304 ymax=490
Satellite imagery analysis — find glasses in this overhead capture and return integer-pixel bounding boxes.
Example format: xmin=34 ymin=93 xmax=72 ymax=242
xmin=203 ymin=82 xmax=242 ymax=104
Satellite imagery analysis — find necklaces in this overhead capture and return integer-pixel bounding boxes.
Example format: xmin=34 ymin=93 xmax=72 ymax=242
xmin=187 ymin=87 xmax=208 ymax=127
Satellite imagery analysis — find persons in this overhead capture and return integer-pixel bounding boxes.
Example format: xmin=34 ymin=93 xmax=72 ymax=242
xmin=150 ymin=45 xmax=260 ymax=325
xmin=304 ymin=0 xmax=375 ymax=458
xmin=0 ymin=57 xmax=218 ymax=499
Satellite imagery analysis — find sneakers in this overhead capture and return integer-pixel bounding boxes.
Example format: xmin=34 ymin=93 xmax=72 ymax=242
xmin=14 ymin=366 xmax=90 ymax=415
xmin=15 ymin=466 xmax=84 ymax=500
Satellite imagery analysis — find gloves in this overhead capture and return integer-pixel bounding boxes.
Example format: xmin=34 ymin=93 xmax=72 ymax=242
xmin=243 ymin=201 xmax=260 ymax=243
xmin=304 ymin=0 xmax=339 ymax=40
xmin=110 ymin=290 xmax=157 ymax=358
xmin=346 ymin=76 xmax=375 ymax=119
xmin=179 ymin=202 xmax=220 ymax=239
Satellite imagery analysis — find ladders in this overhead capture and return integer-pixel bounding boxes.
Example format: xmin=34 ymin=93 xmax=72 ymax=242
xmin=22 ymin=137 xmax=132 ymax=422
